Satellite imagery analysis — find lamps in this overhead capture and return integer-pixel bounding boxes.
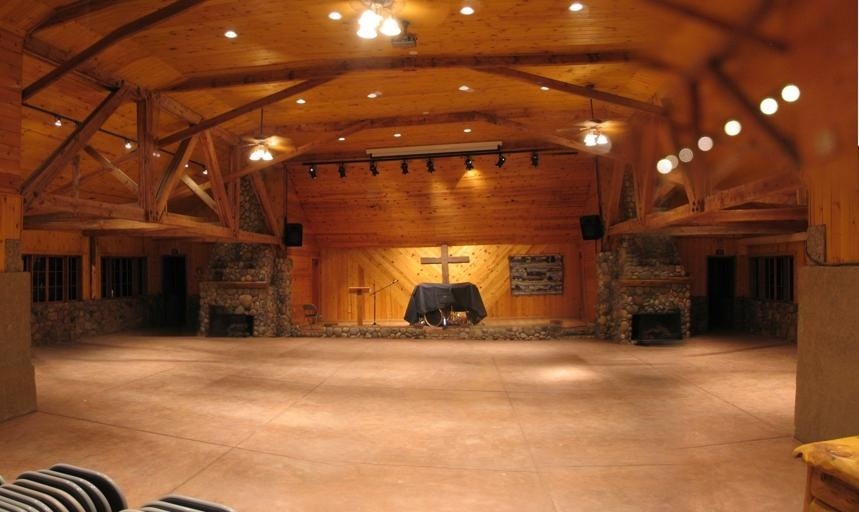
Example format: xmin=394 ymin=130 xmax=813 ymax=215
xmin=356 ymin=0 xmax=401 ymax=40
xmin=581 ymin=83 xmax=609 ymax=148
xmin=250 ymin=106 xmax=272 ymax=163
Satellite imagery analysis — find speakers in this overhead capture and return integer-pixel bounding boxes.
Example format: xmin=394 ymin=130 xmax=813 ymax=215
xmin=581 ymin=215 xmax=602 ymax=240
xmin=285 ymin=223 xmax=303 ymax=246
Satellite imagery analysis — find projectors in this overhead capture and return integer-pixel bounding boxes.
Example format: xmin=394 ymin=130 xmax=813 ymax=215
xmin=392 ymin=35 xmax=416 ymax=47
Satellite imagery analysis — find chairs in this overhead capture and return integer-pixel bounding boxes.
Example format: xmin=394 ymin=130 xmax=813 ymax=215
xmin=0 ymin=462 xmax=235 ymax=512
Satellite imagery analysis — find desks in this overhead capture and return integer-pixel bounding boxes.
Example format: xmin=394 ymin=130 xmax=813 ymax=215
xmin=793 ymin=436 xmax=859 ymax=512
